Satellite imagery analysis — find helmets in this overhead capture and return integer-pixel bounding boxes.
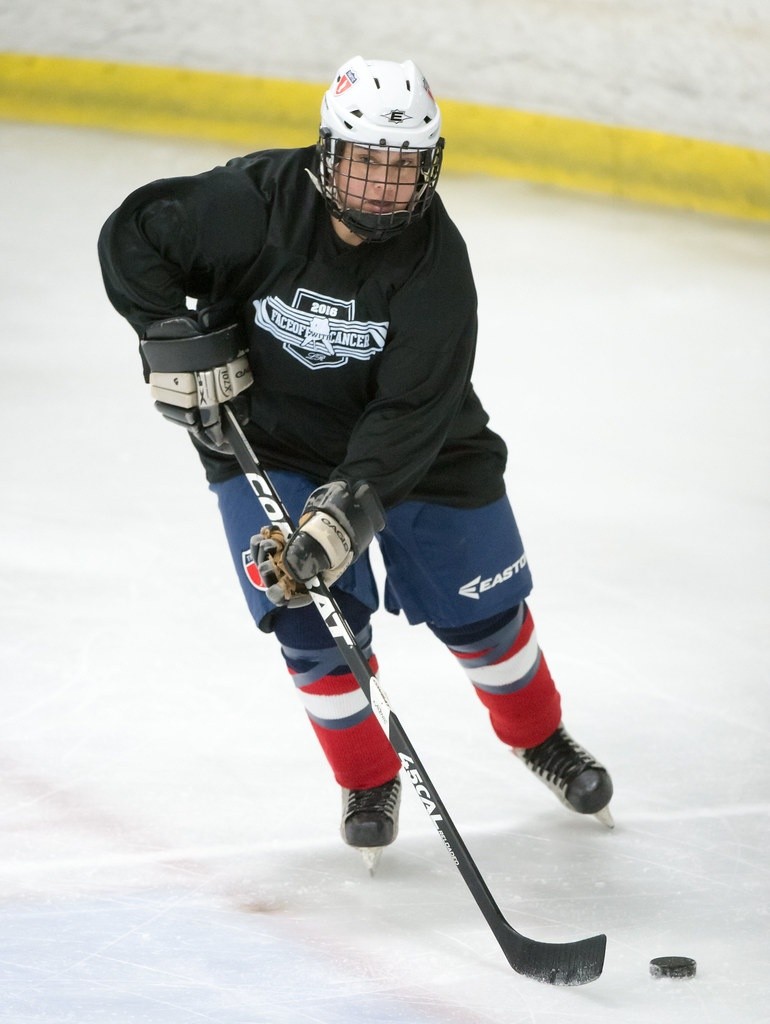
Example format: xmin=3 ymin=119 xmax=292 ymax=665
xmin=319 ymin=56 xmax=444 ymax=247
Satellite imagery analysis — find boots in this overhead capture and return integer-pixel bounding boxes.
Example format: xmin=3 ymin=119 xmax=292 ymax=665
xmin=511 ymin=724 xmax=614 ymax=834
xmin=341 ymin=776 xmax=400 ymax=877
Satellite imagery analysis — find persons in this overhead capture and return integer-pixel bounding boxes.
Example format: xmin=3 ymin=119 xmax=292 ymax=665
xmin=99 ymin=57 xmax=608 ymax=846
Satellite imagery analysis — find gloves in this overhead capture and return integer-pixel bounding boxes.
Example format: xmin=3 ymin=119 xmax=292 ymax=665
xmin=140 ymin=299 xmax=255 ymax=447
xmin=249 ymin=478 xmax=385 ymax=606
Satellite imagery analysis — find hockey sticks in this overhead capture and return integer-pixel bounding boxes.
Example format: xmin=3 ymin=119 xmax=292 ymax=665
xmin=217 ymin=406 xmax=610 ymax=981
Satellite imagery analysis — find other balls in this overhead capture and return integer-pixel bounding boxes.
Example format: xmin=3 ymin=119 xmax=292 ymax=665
xmin=651 ymin=956 xmax=693 ymax=978
